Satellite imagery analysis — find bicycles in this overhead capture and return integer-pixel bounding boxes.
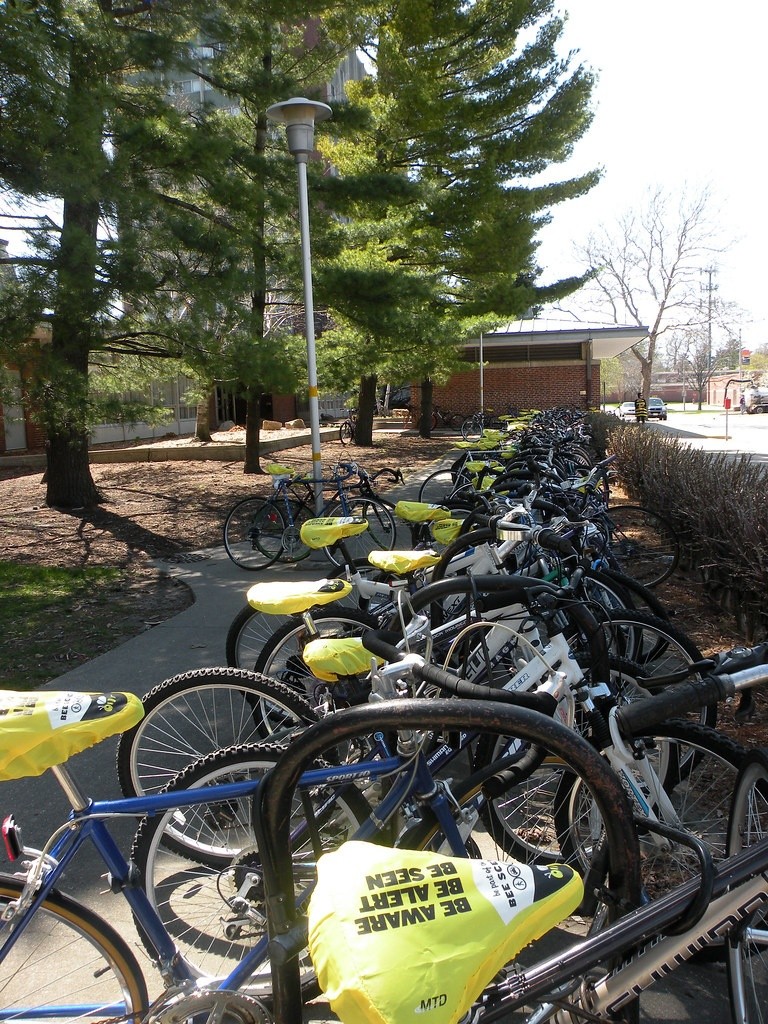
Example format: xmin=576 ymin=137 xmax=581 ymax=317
xmin=1 ymin=403 xmax=768 ymax=1024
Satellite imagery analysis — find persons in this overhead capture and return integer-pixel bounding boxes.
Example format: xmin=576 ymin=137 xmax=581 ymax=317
xmin=635 ymin=393 xmax=647 ymax=426
xmin=740 ymin=395 xmax=747 ymax=415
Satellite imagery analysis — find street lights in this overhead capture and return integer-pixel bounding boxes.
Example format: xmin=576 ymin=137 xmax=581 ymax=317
xmin=260 ymin=93 xmax=338 ymax=561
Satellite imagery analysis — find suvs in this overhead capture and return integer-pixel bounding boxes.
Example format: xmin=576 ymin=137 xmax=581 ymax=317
xmin=643 ymin=397 xmax=668 ymax=422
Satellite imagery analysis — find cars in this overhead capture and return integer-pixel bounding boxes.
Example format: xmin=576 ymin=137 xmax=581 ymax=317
xmin=618 ymin=402 xmax=638 ymax=418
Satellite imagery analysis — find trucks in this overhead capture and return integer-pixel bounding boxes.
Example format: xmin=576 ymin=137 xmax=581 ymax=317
xmin=738 ymin=386 xmax=768 ymax=413
